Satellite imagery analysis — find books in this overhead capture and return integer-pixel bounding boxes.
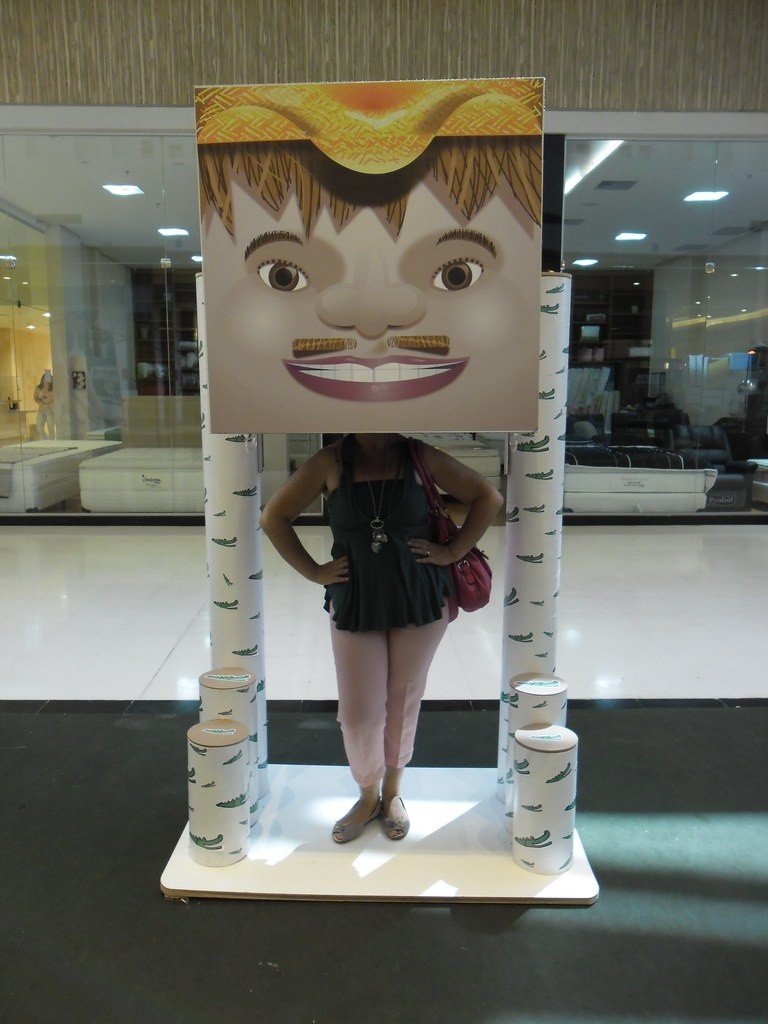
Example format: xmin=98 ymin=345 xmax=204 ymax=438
xmin=181 ymin=373 xmax=199 ymax=385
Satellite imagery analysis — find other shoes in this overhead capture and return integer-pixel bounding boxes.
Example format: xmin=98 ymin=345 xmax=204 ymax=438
xmin=381 ymin=797 xmax=410 ymax=840
xmin=331 ymin=796 xmax=381 ymax=843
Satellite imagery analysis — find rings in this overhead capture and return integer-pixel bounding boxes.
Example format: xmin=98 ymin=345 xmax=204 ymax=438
xmin=427 ymin=551 xmax=430 ymax=555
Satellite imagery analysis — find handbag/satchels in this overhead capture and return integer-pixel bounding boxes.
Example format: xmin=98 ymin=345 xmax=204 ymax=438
xmin=407 ymin=436 xmax=492 ymax=624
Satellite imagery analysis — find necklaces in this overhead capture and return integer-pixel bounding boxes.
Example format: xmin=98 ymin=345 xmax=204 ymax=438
xmin=355 ymin=441 xmax=391 ymax=553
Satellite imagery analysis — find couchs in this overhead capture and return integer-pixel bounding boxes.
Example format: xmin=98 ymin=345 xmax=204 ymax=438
xmin=666 ymin=424 xmax=757 ymax=512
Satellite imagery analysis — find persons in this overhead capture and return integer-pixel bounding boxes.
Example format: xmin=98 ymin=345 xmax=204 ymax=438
xmin=33 ymin=374 xmax=55 ymax=441
xmin=260 ymin=432 xmax=504 ymax=844
